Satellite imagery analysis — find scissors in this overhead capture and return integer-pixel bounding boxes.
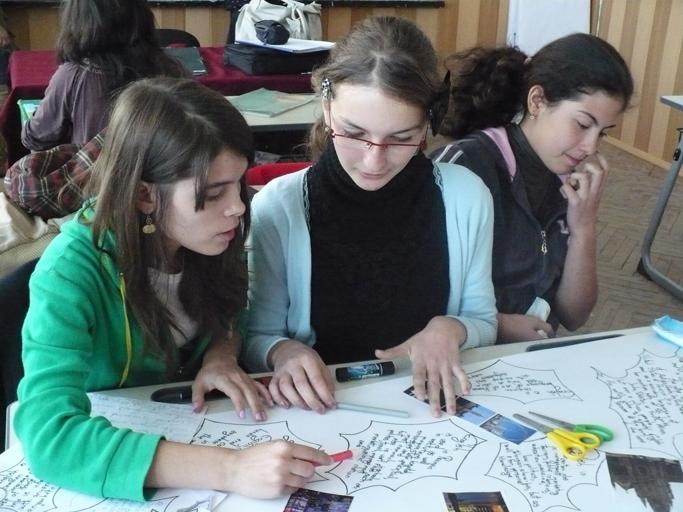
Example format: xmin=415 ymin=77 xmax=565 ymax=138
xmin=513 ymin=413 xmax=601 ymax=460
xmin=528 ymin=411 xmax=613 ymax=443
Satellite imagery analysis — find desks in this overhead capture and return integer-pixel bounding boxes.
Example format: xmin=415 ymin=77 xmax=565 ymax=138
xmin=4 ymin=314 xmax=683 ymax=512
xmin=6 ymin=43 xmax=319 ymax=173
xmin=18 ymin=91 xmax=325 ymax=172
xmin=637 ymin=92 xmax=683 ymax=304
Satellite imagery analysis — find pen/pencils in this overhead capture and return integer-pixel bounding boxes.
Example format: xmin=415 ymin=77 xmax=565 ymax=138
xmin=336 ymin=403 xmax=409 ymax=419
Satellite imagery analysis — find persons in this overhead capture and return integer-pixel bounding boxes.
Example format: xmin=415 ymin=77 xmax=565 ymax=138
xmin=14 ymin=76 xmax=333 ymax=503
xmin=21 ymin=0 xmax=189 ymax=149
xmin=425 ymin=31 xmax=638 ymax=345
xmin=243 ymin=16 xmax=498 ymax=419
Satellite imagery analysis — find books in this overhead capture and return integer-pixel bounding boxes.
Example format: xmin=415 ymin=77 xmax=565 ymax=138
xmin=226 ymin=86 xmax=317 ymax=118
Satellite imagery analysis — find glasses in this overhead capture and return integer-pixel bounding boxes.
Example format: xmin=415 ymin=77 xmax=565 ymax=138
xmin=329 ymin=98 xmax=430 ymax=155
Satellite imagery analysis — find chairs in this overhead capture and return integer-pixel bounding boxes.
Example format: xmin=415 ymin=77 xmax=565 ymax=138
xmin=243 ymin=159 xmax=318 ymax=186
xmin=153 ymin=28 xmax=199 ymax=47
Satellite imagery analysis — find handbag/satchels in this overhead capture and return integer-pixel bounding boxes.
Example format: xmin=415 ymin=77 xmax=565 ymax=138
xmin=223 ymin=44 xmax=330 ymax=77
xmin=233 ymin=0 xmax=322 ymax=43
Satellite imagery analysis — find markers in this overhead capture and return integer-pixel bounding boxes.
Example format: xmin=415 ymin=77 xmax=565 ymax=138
xmin=315 ymin=450 xmax=353 ymax=466
